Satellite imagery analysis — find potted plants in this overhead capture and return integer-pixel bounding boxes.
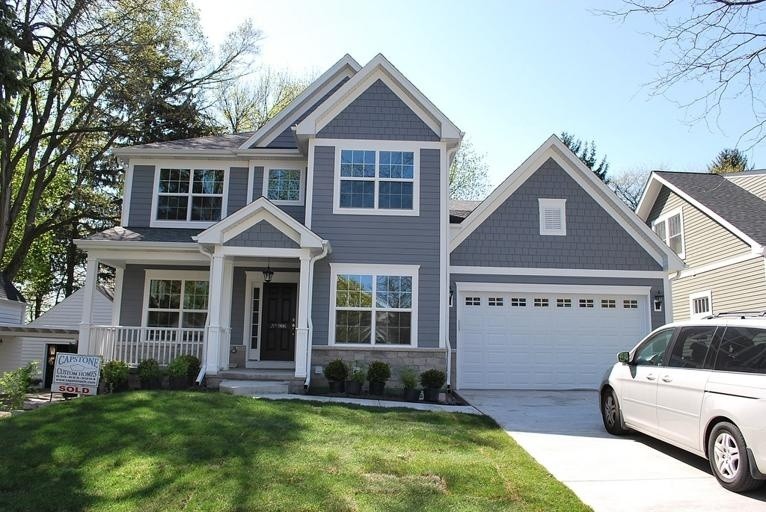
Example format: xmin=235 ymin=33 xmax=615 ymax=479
xmin=323 ymin=359 xmax=445 ymax=402
xmin=101 ymin=354 xmax=201 ymax=392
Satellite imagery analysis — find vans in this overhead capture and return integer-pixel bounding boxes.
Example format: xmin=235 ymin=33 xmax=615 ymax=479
xmin=598 ymin=308 xmax=766 ymax=492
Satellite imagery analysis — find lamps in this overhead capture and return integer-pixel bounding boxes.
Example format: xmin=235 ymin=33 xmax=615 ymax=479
xmin=262 ymin=258 xmax=274 ymax=283
